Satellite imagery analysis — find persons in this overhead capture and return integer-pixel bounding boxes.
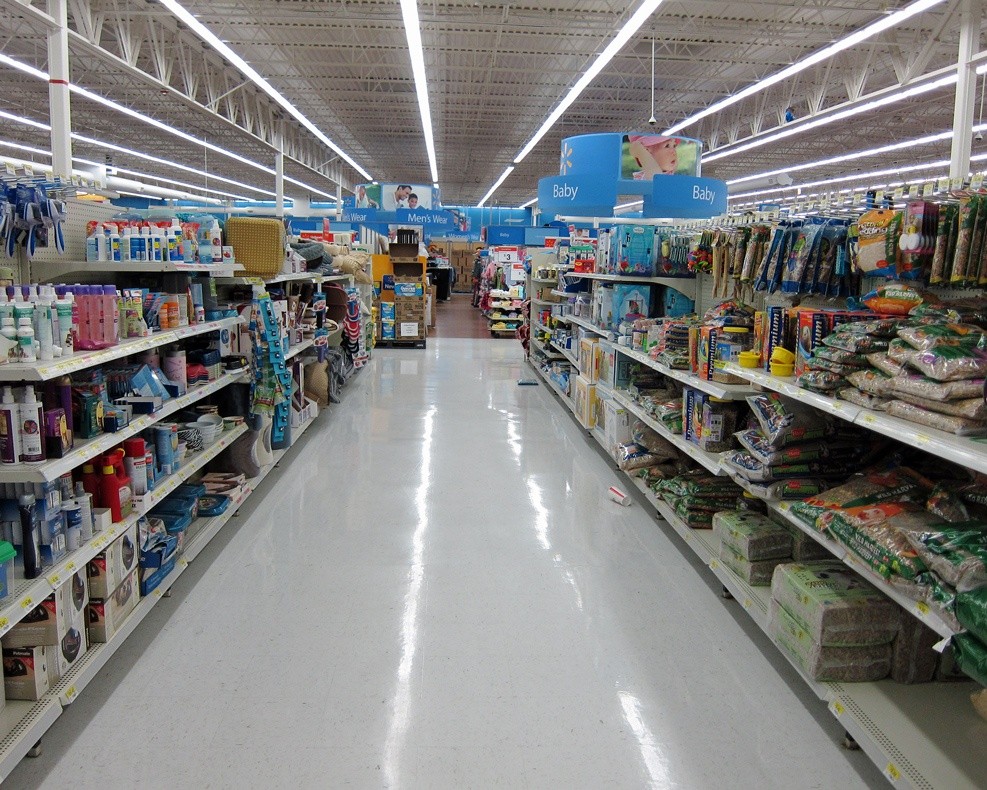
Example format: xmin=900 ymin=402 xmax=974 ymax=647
xmin=392 ymin=185 xmax=418 ymax=211
xmin=357 ymin=186 xmax=368 ymax=208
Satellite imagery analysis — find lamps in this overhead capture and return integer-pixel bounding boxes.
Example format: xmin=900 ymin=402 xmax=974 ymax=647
xmin=0 ymin=0 xmax=987 ymax=200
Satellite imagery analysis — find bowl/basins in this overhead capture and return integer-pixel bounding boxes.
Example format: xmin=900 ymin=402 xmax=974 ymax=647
xmin=771 ymin=346 xmax=795 ymax=364
xmin=737 ymin=355 xmax=760 ymax=368
xmin=769 ymin=362 xmax=795 ymax=377
xmin=176 ymin=402 xmax=244 ymax=464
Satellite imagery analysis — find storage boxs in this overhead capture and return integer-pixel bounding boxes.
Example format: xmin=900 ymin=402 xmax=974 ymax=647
xmin=113 ymin=395 xmax=164 ymax=414
xmin=449 ymin=247 xmax=474 ymax=293
xmin=571 ymin=221 xmax=879 ymax=451
xmin=207 ymin=327 xmax=235 ymax=359
xmin=4 ymin=520 xmax=143 ymax=711
xmin=220 ymin=213 xmax=288 ymax=282
xmin=393 ymin=282 xmax=429 ymax=341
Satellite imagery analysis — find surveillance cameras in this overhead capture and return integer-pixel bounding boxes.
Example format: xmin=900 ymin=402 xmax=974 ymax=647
xmin=975 ymin=134 xmax=983 ymax=142
xmin=648 ymin=116 xmax=657 ymax=125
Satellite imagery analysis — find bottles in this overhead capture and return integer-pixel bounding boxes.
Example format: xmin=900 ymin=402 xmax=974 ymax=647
xmin=198 ymin=239 xmax=213 ymax=264
xmin=95 ymin=218 xmax=185 ymax=264
xmin=712 ymin=326 xmax=752 ymax=385
xmin=0 ymin=385 xmax=48 ymax=466
xmin=0 ymin=283 xmax=120 ymax=363
xmin=209 ymin=218 xmax=223 ymax=261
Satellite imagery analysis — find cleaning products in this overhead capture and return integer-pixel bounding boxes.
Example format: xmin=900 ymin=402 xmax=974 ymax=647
xmin=81 ymin=448 xmax=136 ymax=524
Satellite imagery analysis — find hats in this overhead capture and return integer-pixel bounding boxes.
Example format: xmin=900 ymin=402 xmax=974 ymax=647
xmin=628 ymin=134 xmax=680 ymax=149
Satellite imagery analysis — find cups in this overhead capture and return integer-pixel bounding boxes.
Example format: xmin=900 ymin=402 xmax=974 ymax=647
xmin=608 ymin=486 xmax=632 ymax=506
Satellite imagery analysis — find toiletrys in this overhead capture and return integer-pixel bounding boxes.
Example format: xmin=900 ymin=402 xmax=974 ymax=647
xmin=0 ymin=282 xmax=205 ymax=364
xmin=0 ymin=386 xmax=46 ymax=466
xmin=18 ymin=494 xmax=43 ymax=579
xmin=86 ymin=216 xmax=224 ymax=263
xmin=61 ymin=481 xmax=95 ymax=551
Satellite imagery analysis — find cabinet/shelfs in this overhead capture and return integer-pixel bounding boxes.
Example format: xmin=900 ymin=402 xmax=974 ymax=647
xmin=0 ymin=253 xmax=374 ymax=790
xmin=474 ymin=238 xmax=524 ymax=335
xmin=525 ymin=213 xmax=987 ymax=790
